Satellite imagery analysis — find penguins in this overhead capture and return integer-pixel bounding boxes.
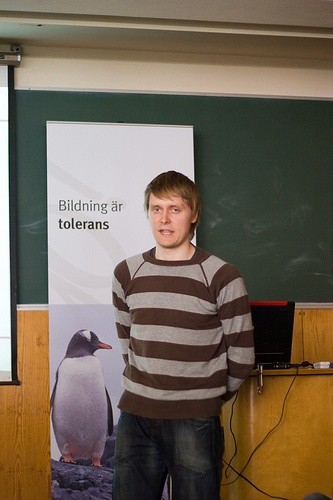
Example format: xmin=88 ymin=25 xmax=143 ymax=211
xmin=50 ymin=329 xmax=114 ymax=468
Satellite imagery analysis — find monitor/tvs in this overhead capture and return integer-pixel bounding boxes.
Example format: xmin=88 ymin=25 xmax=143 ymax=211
xmin=249 ymin=301 xmax=296 ymax=368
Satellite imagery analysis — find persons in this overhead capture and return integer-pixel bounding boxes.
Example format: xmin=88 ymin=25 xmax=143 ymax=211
xmin=112 ymin=169 xmax=258 ymax=500
